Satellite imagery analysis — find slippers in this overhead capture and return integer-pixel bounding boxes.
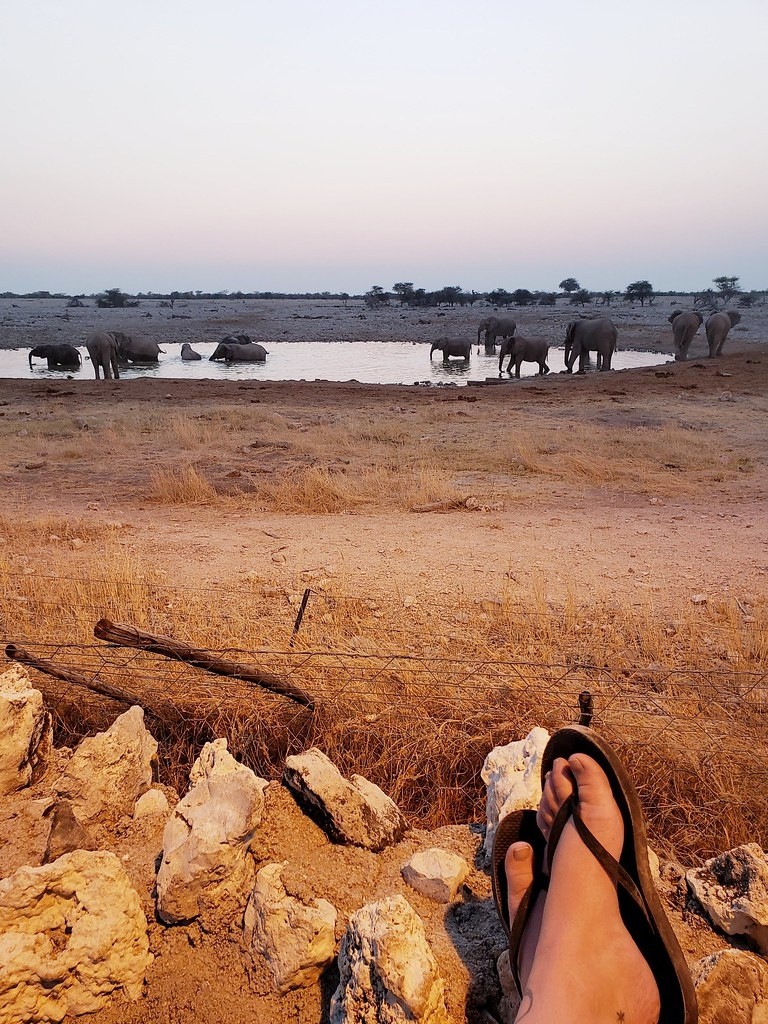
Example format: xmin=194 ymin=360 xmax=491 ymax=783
xmin=541 ymin=726 xmax=699 ymax=1024
xmin=490 ymin=810 xmax=547 ymax=1002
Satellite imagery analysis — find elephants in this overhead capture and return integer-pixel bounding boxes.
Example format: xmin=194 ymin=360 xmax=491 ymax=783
xmin=29 ymin=331 xmax=269 ymax=379
xmin=430 ymin=316 xmax=619 ymax=378
xmin=669 ymin=310 xmax=741 ymax=361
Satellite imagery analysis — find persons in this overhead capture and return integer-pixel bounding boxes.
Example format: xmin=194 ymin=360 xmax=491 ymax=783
xmin=491 ymin=724 xmax=698 ymax=1024
